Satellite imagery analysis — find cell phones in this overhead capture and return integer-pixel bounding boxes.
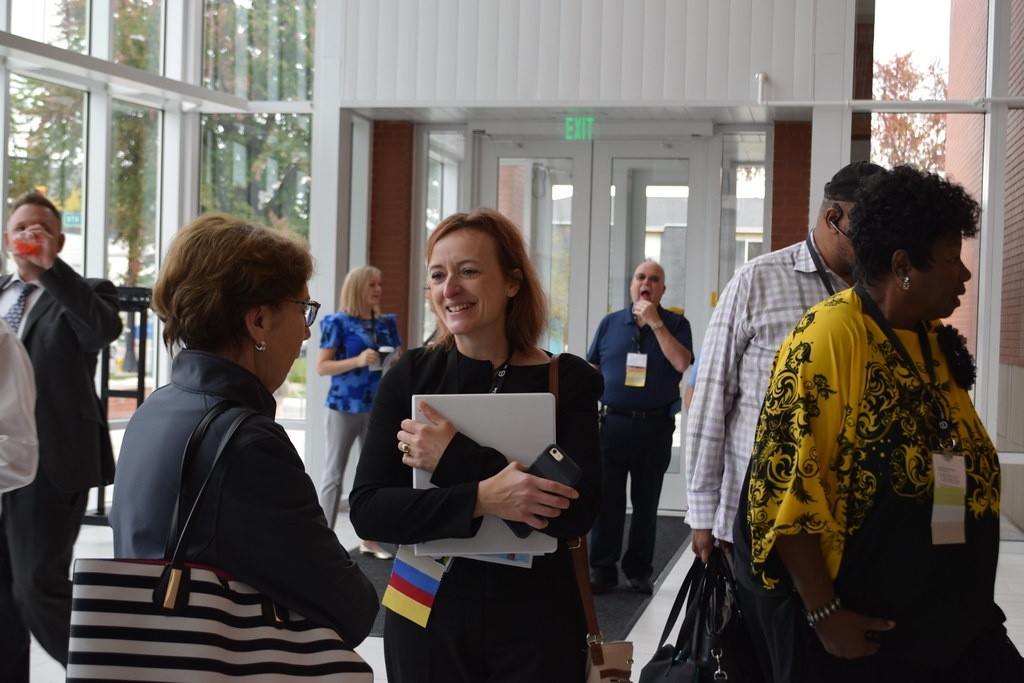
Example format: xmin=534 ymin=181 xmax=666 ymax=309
xmin=504 ymin=444 xmax=584 ymax=537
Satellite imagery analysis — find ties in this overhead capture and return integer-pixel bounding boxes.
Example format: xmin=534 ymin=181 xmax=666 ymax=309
xmin=3 ymin=280 xmax=39 ymax=336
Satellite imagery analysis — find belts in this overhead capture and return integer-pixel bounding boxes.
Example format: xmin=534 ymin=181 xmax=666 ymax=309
xmin=606 ymin=407 xmax=669 ymax=419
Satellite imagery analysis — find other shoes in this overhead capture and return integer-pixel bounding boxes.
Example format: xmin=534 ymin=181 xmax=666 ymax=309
xmin=360 ymin=543 xmax=393 ymax=559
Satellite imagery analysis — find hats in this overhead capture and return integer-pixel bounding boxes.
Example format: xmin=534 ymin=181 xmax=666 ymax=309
xmin=823 ymin=160 xmax=889 ymax=202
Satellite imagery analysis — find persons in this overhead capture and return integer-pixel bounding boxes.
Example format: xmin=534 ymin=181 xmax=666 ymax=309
xmin=586 ymin=261 xmax=695 ymax=594
xmin=683 ymin=354 xmax=700 ymax=412
xmin=110 ymin=212 xmax=379 ymax=649
xmin=0 ymin=190 xmax=125 ymax=683
xmin=683 ymin=160 xmax=889 ymax=564
xmin=349 ymin=210 xmax=605 ymax=683
xmin=732 ymin=165 xmax=1000 ymax=683
xmin=315 ymin=267 xmax=403 ymax=559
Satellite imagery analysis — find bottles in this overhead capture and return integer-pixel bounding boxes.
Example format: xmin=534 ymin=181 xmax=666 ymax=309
xmin=11 ymin=231 xmax=38 ymax=255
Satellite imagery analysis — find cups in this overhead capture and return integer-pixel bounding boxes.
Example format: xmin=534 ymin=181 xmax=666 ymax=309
xmin=378 ymin=346 xmax=394 ymax=367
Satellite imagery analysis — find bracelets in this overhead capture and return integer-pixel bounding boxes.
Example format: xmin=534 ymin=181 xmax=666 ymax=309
xmin=803 ymin=595 xmax=843 ymax=624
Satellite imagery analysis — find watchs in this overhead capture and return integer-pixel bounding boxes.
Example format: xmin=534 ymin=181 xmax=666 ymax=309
xmin=649 ymin=320 xmax=664 ymax=329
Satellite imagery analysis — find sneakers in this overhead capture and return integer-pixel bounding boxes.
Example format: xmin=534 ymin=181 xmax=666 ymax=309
xmin=588 ymin=564 xmax=618 ymax=592
xmin=632 ymin=576 xmax=653 ymax=594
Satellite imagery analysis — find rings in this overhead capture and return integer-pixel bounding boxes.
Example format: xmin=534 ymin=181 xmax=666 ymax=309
xmin=402 ymin=443 xmax=411 ymax=455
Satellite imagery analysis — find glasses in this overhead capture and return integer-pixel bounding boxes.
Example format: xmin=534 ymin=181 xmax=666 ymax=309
xmin=277 ymin=300 xmax=321 ymax=327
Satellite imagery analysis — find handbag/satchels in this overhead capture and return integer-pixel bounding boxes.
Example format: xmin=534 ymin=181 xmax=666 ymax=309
xmin=641 ymin=546 xmax=703 ymax=683
xmin=65 ymin=404 xmax=375 ymax=683
xmin=585 ymin=639 xmax=633 ymax=683
xmin=699 ymin=547 xmax=761 ymax=683
